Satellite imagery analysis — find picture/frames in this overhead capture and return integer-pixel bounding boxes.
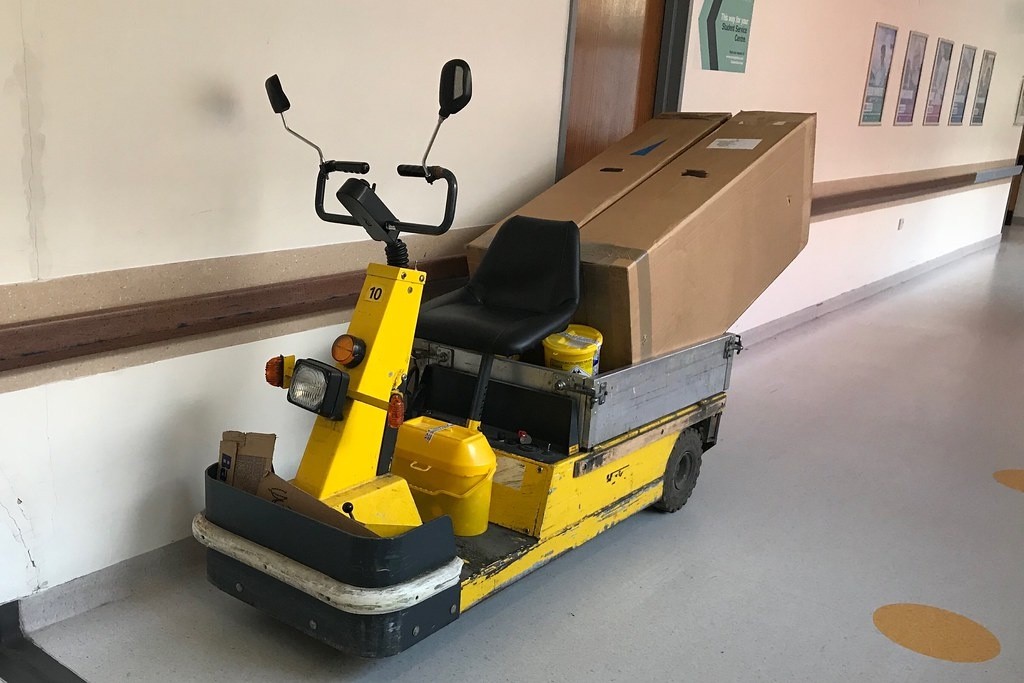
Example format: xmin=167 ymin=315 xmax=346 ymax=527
xmin=948 ymin=44 xmax=977 ymax=125
xmin=1014 ymin=77 xmax=1024 ymax=125
xmin=922 ymin=38 xmax=954 ymax=126
xmin=893 ymin=31 xmax=929 ymax=126
xmin=858 ymin=22 xmax=898 ymax=127
xmin=970 ymin=50 xmax=997 ymax=125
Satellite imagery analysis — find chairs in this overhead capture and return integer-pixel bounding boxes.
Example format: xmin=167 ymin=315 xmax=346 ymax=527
xmin=419 ymin=211 xmax=580 ymax=418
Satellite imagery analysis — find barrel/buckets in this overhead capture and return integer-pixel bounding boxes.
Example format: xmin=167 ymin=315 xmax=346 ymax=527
xmin=542 ymin=323 xmax=605 ymax=377
xmin=388 ymin=415 xmax=496 ymax=536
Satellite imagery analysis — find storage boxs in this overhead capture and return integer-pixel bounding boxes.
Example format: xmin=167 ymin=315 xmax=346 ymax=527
xmin=465 ymin=109 xmax=818 ymax=367
